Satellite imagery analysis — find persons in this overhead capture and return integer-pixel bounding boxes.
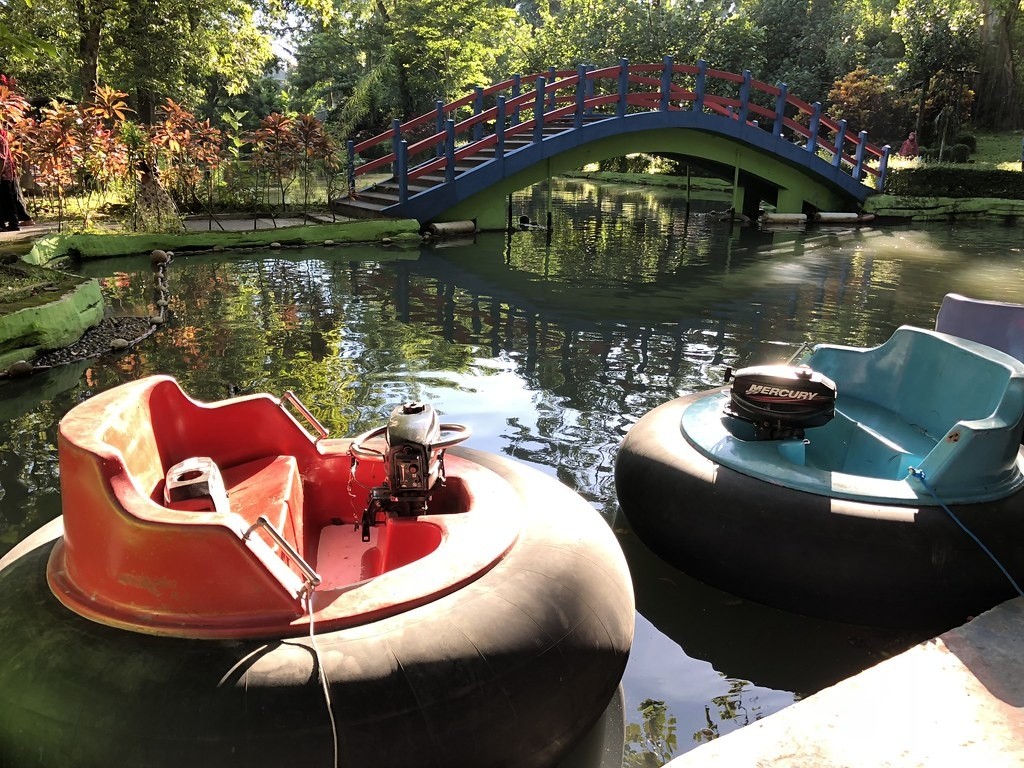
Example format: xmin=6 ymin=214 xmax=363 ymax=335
xmin=0 ymin=129 xmax=35 ymax=232
xmin=899 ymin=132 xmax=919 ymax=161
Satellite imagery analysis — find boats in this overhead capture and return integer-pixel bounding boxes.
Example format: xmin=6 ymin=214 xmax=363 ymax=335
xmin=607 ymin=293 xmax=1024 ymax=630
xmin=0 ymin=371 xmax=637 ymax=768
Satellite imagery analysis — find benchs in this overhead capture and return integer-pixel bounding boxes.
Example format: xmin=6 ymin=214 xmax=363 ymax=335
xmin=797 ymin=323 xmax=1024 ymax=502
xmin=936 ymin=293 xmax=1024 ymax=361
xmin=55 ymin=376 xmax=322 ymax=625
